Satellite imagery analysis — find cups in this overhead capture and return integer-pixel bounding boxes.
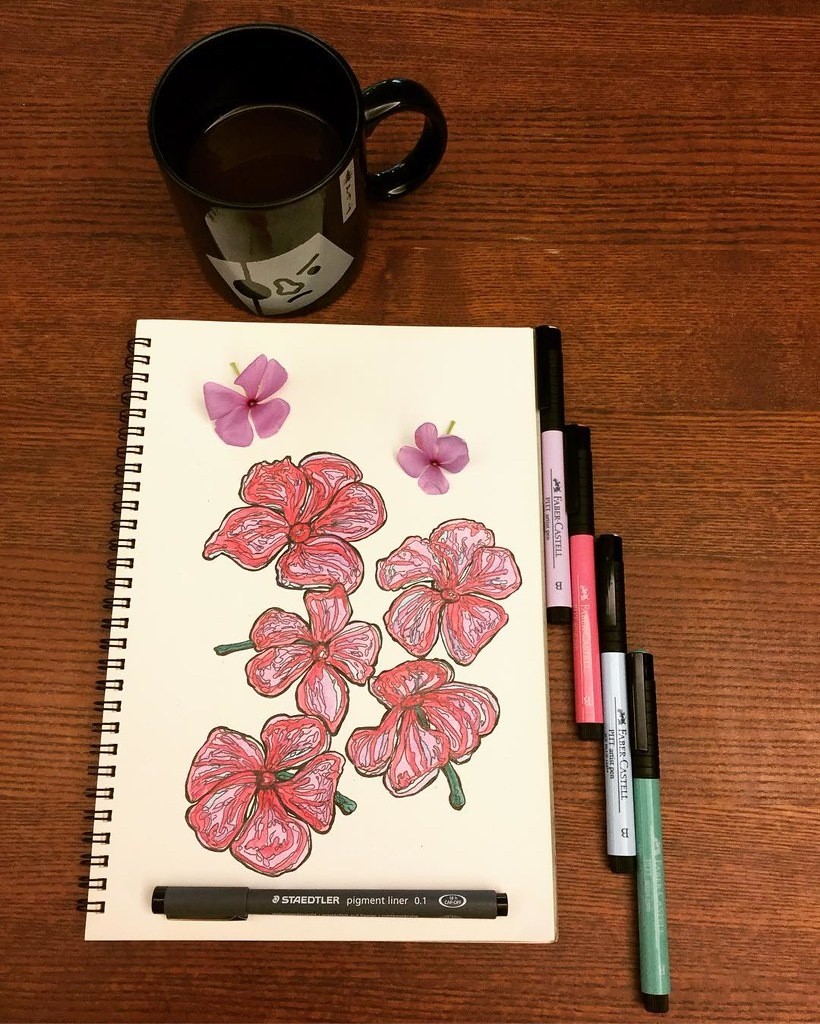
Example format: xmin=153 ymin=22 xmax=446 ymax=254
xmin=147 ymin=25 xmax=449 ymax=318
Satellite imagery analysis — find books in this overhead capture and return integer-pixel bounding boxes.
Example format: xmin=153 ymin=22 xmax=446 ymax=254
xmin=76 ymin=317 xmax=559 ymax=948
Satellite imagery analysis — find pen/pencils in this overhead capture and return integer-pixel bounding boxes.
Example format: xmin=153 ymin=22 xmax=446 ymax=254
xmin=627 ymin=650 xmax=671 ymax=1013
xmin=562 ymin=423 xmax=604 ymax=738
xmin=593 ymin=533 xmax=637 ymax=874
xmin=532 ymin=324 xmax=571 ymax=623
xmin=150 ymin=885 xmax=509 ymax=922
